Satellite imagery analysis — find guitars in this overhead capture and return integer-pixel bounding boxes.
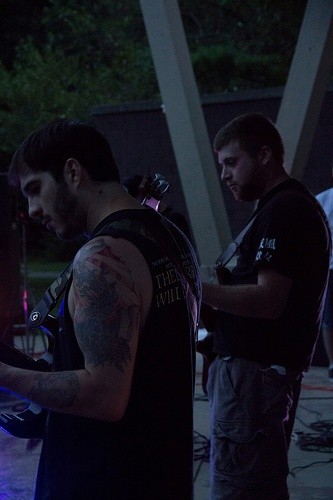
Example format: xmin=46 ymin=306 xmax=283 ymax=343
xmin=0 ymin=296 xmax=61 ymax=440
xmin=193 ymin=259 xmax=232 ymax=397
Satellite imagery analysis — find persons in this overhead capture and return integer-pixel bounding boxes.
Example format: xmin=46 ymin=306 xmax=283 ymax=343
xmin=0 ymin=119 xmax=202 ymax=500
xmin=202 ymin=112 xmax=332 ymax=500
